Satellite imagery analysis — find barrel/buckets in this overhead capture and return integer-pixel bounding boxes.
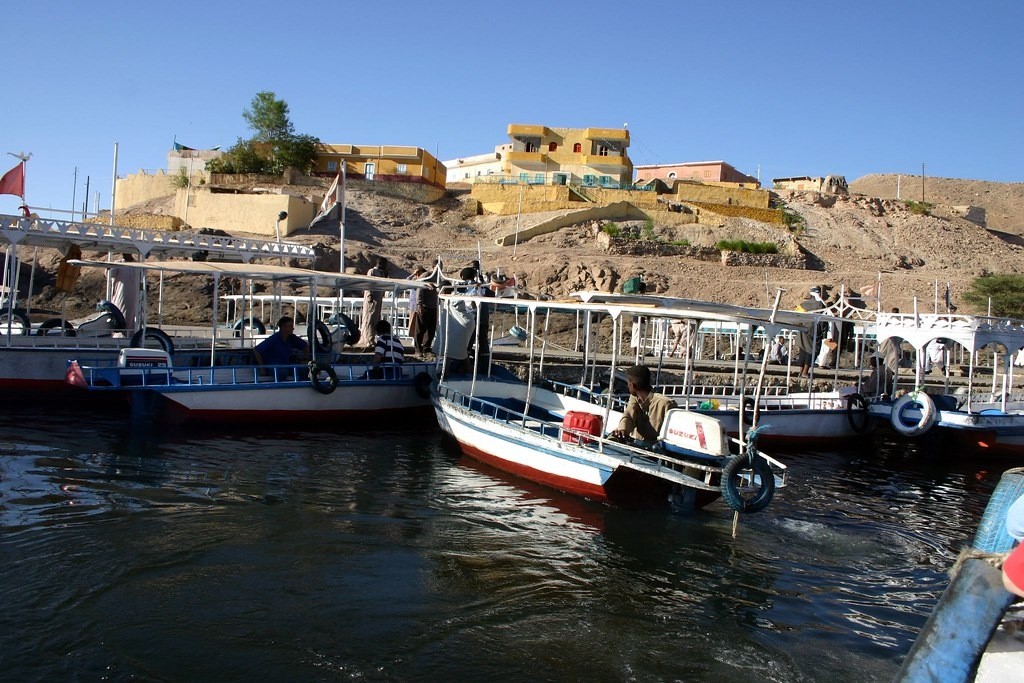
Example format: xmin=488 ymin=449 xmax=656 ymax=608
xmin=561 ymin=411 xmax=604 ymax=444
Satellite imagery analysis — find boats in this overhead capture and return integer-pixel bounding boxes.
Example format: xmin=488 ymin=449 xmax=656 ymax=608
xmin=892 ymin=466 xmax=1024 ymax=683
xmin=0 ymin=152 xmax=346 ymax=390
xmin=66 ymin=258 xmax=527 ymax=421
xmin=566 ymin=278 xmax=1024 ymax=455
xmin=430 ymin=285 xmax=788 ymax=539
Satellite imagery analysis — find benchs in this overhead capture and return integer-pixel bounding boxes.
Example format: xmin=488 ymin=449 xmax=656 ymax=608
xmin=514 ymin=395 xmax=569 ymax=437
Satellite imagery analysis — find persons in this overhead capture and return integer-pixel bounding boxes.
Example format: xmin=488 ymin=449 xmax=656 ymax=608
xmin=357 ymin=320 xmax=403 ymax=380
xmin=406 ymin=260 xmax=495 ymax=374
xmin=795 ymin=287 xmax=854 ymax=377
xmin=359 ymin=258 xmax=390 ymax=346
xmin=254 ymin=316 xmax=308 ymax=380
xmin=105 ymin=252 xmax=141 ymax=333
xmin=912 ymin=340 xmax=945 ymax=375
xmin=854 ymin=351 xmax=893 ymax=402
xmin=604 ymin=365 xmax=684 ymax=473
xmin=631 ymin=283 xmax=647 ymax=355
xmin=732 ymin=347 xmax=745 ymax=360
xmin=668 ymin=324 xmax=695 ymax=359
xmin=758 ymin=349 xmax=764 ymax=360
xmin=771 ymin=337 xmax=788 ymax=364
xmin=1002 ymin=493 xmax=1024 ymax=599
xmin=880 ymin=307 xmax=901 ymax=374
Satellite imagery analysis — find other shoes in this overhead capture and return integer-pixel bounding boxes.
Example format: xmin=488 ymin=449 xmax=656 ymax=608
xmin=422 ymin=351 xmax=436 ymax=358
xmin=414 ymin=350 xmax=423 ymax=357
xmin=797 ymin=373 xmax=810 ymax=378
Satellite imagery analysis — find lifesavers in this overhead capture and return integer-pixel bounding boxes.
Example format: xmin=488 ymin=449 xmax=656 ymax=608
xmin=740 ymin=397 xmax=760 ymax=424
xmin=233 ymin=316 xmax=267 ymax=336
xmin=329 ymin=313 xmax=360 ymax=345
xmin=95 ymin=299 xmax=126 ymax=331
xmin=307 ymin=319 xmax=332 ymax=353
xmin=35 ymin=318 xmax=76 ymax=336
xmin=130 ymin=326 xmax=175 ymax=356
xmin=0 ymin=307 xmax=31 ymax=336
xmin=721 ymin=453 xmax=775 ymax=514
xmin=891 ymin=391 xmax=937 ymax=436
xmin=847 ymin=393 xmax=868 ymax=432
xmin=310 ymin=363 xmax=339 ymax=394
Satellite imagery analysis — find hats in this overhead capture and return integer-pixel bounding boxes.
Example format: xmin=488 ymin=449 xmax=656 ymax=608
xmin=867 ymin=351 xmax=885 ymax=359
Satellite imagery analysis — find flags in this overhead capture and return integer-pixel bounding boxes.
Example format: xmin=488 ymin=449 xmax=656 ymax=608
xmin=944 ymin=288 xmax=957 ymax=312
xmin=310 ymin=172 xmax=342 ymax=227
